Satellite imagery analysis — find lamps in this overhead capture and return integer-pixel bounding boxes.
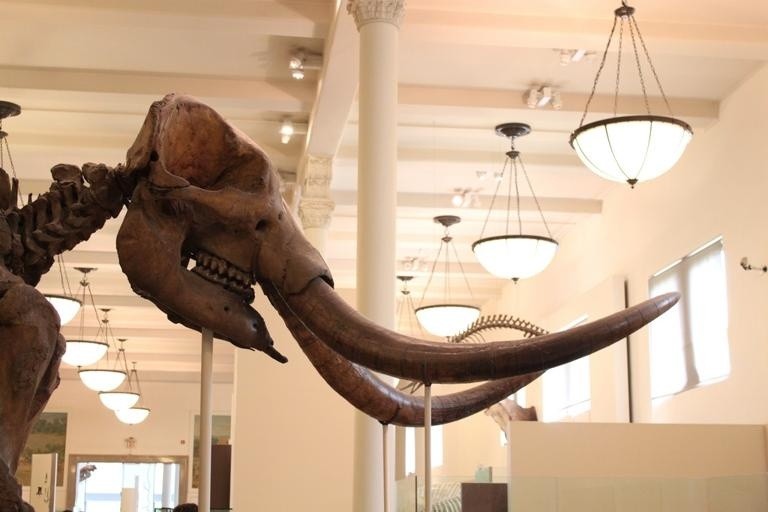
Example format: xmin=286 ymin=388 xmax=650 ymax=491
xmin=41 ymin=265 xmax=151 ymax=427
xmin=288 ymin=48 xmax=322 ymax=82
xmin=414 ymin=124 xmax=558 ymax=341
xmin=569 ymin=2 xmax=694 ymax=190
xmin=280 ymin=117 xmax=308 ymax=146
